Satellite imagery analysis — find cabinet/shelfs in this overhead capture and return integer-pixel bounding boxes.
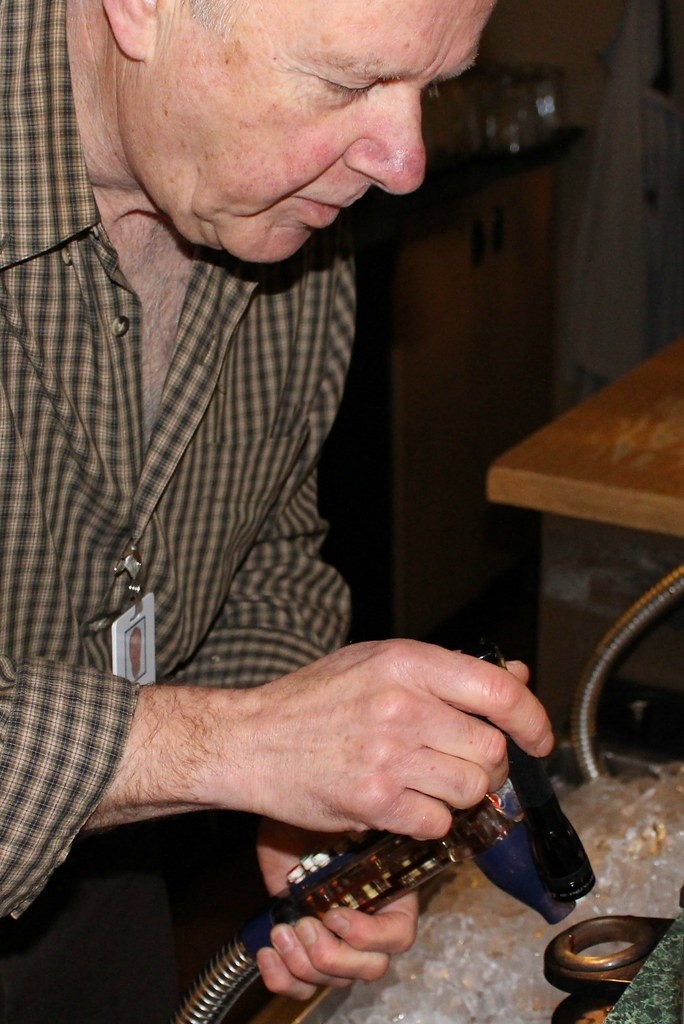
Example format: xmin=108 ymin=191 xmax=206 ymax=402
xmin=315 ymin=163 xmax=555 ymax=647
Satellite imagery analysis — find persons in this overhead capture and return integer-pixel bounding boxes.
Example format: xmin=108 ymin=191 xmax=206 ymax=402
xmin=0 ymin=0 xmax=555 ymax=1024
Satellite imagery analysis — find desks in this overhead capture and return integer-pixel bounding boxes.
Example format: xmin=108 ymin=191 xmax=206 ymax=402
xmin=486 ymin=334 xmax=684 ymax=741
